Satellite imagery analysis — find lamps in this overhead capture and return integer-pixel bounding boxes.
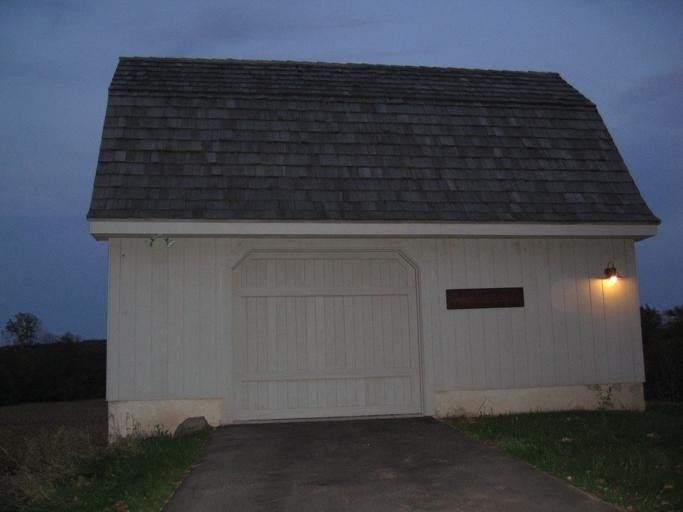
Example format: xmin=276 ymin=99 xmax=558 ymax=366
xmin=604 ymin=261 xmax=624 ymax=286
